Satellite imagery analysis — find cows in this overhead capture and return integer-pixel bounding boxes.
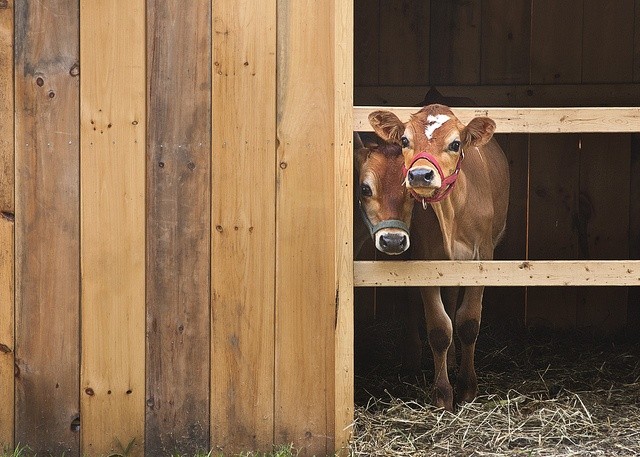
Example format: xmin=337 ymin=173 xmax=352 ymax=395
xmin=354 ymin=131 xmax=422 ymax=405
xmin=368 ymin=104 xmax=511 ymax=412
